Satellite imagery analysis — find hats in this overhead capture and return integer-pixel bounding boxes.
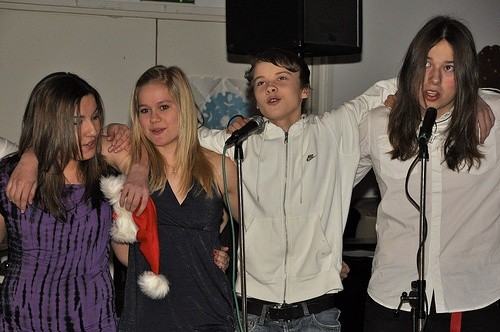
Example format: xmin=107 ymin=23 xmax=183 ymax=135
xmin=100 ymin=174 xmax=169 ymax=300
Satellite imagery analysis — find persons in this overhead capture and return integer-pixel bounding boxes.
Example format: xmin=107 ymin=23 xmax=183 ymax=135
xmin=196 ymin=44 xmax=496 ymax=332
xmin=0 ymin=72 xmax=231 ymax=332
xmin=352 ymin=16 xmax=500 ymax=332
xmin=0 ymin=122 xmax=132 ymax=159
xmin=6 ymin=65 xmax=350 ymax=332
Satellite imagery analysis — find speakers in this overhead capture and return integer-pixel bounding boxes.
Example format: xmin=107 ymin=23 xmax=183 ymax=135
xmin=225 ymin=0 xmax=363 ymax=59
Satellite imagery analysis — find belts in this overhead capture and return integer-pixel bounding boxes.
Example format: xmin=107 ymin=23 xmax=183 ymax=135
xmin=232 ymin=294 xmax=336 ymax=323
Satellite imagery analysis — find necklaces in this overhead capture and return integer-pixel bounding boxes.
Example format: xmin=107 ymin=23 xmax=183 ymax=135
xmin=169 ymin=165 xmax=177 ymax=176
xmin=420 ymin=115 xmax=453 ymax=132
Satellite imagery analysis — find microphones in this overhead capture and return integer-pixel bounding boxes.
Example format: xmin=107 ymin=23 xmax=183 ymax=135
xmin=420 ymin=106 xmax=438 ymax=147
xmin=225 ymin=115 xmax=265 ymax=146
